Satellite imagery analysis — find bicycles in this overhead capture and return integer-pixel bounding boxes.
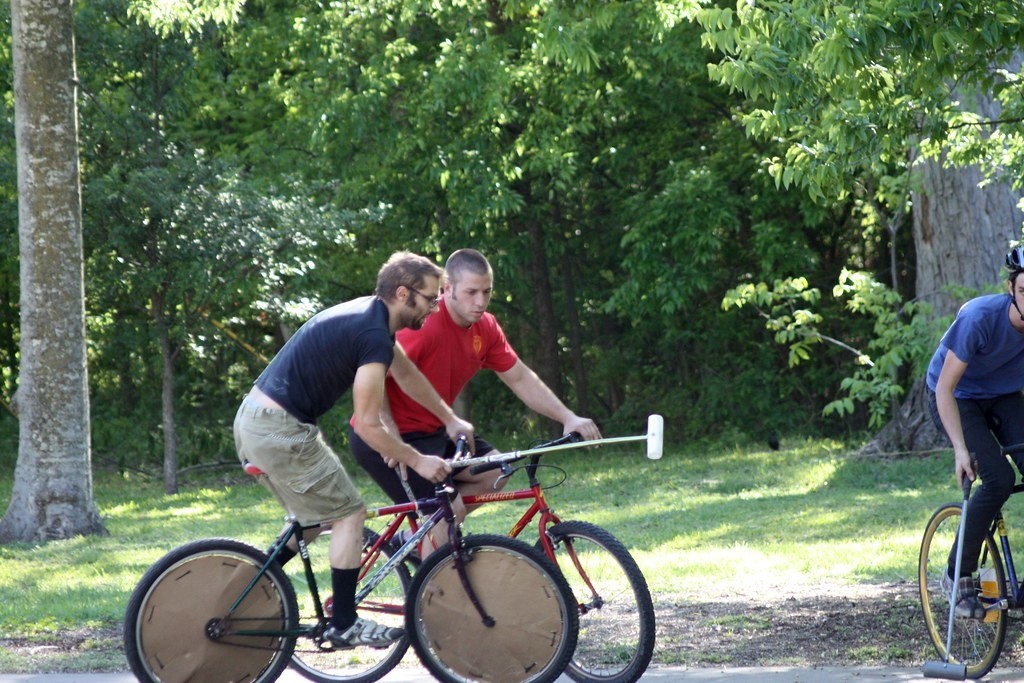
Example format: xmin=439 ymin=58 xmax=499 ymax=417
xmin=265 ymin=429 xmax=657 ymax=683
xmin=120 ymin=435 xmax=579 ymax=683
xmin=918 ymin=415 xmax=1024 ymax=683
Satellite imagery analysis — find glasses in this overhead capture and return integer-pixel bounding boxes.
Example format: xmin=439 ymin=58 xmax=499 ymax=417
xmin=395 ymin=282 xmax=441 ymax=308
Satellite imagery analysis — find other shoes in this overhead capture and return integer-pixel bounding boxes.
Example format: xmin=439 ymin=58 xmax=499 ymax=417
xmin=940 ymin=567 xmax=986 ymax=619
xmin=390 ymin=531 xmax=422 ymax=564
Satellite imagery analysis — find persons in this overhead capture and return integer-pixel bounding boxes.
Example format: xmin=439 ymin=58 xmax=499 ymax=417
xmin=926 ymin=248 xmax=1024 ymax=618
xmin=234 ymin=250 xmax=475 ymax=645
xmin=350 ymin=250 xmax=603 ymax=561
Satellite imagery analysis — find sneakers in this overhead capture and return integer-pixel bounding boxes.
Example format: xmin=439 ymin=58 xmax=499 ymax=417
xmin=327 ymin=617 xmax=403 ymax=650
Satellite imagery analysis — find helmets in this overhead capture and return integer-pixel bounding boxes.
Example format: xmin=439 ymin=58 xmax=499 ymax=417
xmin=1005 ymin=246 xmax=1023 ymax=271
